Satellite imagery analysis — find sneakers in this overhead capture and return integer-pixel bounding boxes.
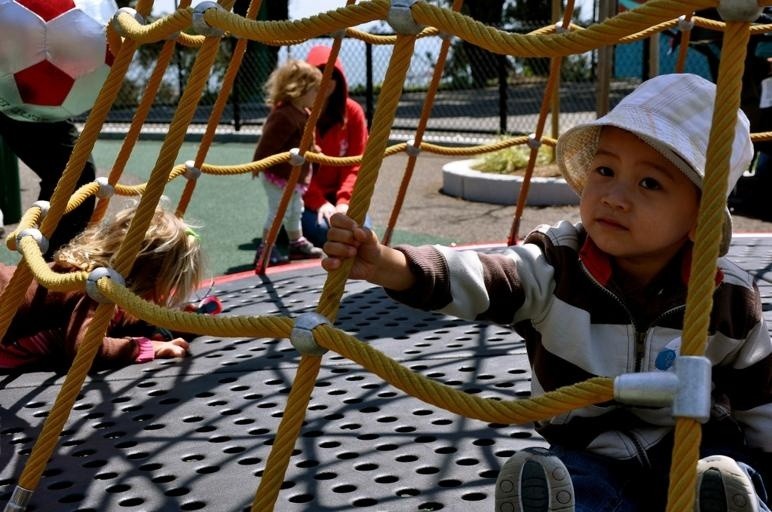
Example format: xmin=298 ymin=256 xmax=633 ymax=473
xmin=287 ymin=234 xmax=325 ymax=261
xmin=252 ymin=242 xmax=291 ymax=265
xmin=494 ymin=446 xmax=577 ymax=510
xmin=692 ymin=456 xmax=757 ymax=510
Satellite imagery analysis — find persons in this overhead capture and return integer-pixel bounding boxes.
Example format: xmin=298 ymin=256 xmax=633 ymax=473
xmin=0 ymin=196 xmax=221 ymax=369
xmin=252 ymin=45 xmax=372 ymax=264
xmin=320 ymin=72 xmax=772 ymax=512
xmin=0 ymin=118 xmax=97 ymax=262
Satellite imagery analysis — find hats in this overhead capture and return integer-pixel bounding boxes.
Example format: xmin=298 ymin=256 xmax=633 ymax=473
xmin=554 ymin=71 xmax=755 ymax=261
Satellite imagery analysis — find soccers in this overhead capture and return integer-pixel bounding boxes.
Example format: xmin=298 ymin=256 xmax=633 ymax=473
xmin=0 ymin=0 xmax=121 ymax=125
